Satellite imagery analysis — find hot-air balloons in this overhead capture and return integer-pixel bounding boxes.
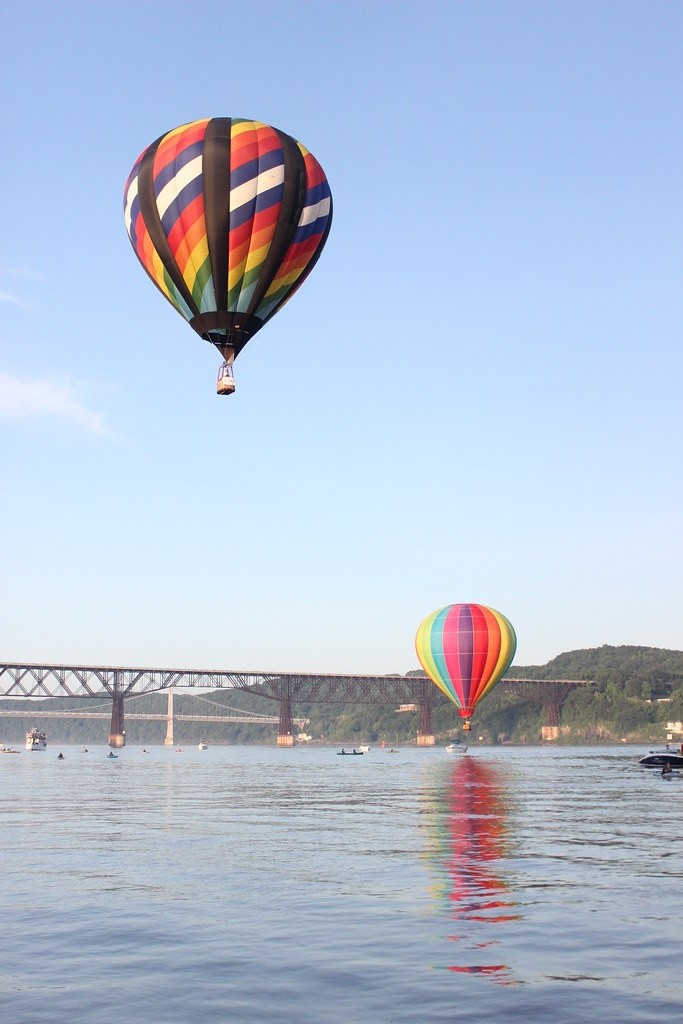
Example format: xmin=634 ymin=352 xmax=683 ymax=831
xmin=414 ymin=603 xmax=518 ymax=731
xmin=119 ymin=114 xmax=336 ymax=399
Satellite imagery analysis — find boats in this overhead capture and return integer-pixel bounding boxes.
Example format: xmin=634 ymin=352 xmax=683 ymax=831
xmin=108 ymin=754 xmax=117 ymax=758
xmin=445 ymin=742 xmax=468 ymax=753
xmin=338 ymin=752 xmax=363 ymax=754
xmin=25 ymin=727 xmax=49 ymax=751
xmin=357 ymin=745 xmax=370 ymax=752
xmin=662 ymin=770 xmax=683 ymax=780
xmin=3 ymin=749 xmax=20 ymax=753
xmin=198 ymin=741 xmax=208 ymax=750
xmin=637 ymin=747 xmax=683 ymax=769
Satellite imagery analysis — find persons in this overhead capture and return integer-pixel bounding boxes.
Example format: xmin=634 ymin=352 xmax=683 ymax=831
xmin=662 ymin=762 xmax=672 ymax=774
